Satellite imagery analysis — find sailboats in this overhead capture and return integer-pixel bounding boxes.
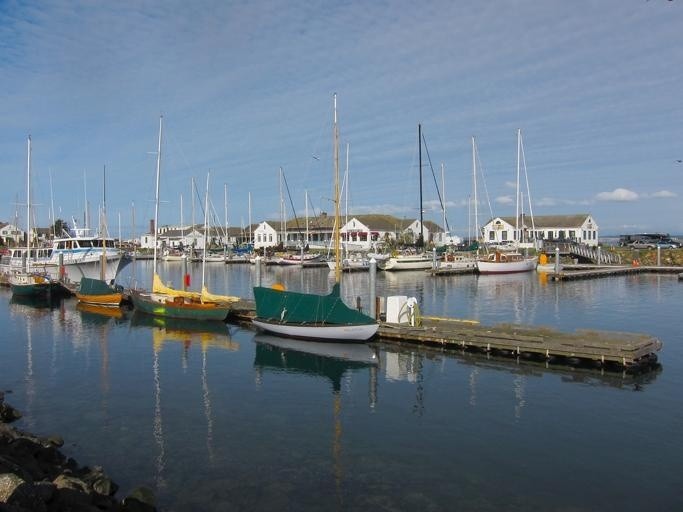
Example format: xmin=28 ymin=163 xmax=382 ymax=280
xmin=250 ymin=92 xmax=380 ymax=340
xmin=130 ymin=116 xmax=240 ymax=322
xmin=130 ymin=171 xmax=250 ymax=264
xmin=0 ymin=134 xmax=135 ymax=312
xmin=250 ymin=142 xmax=441 ymax=272
xmin=426 ymin=129 xmax=539 ymax=273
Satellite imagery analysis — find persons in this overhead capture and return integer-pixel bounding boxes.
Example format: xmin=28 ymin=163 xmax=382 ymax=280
xmin=272 ymin=279 xmax=288 ymax=290
xmin=539 ymin=249 xmax=547 ymax=265
